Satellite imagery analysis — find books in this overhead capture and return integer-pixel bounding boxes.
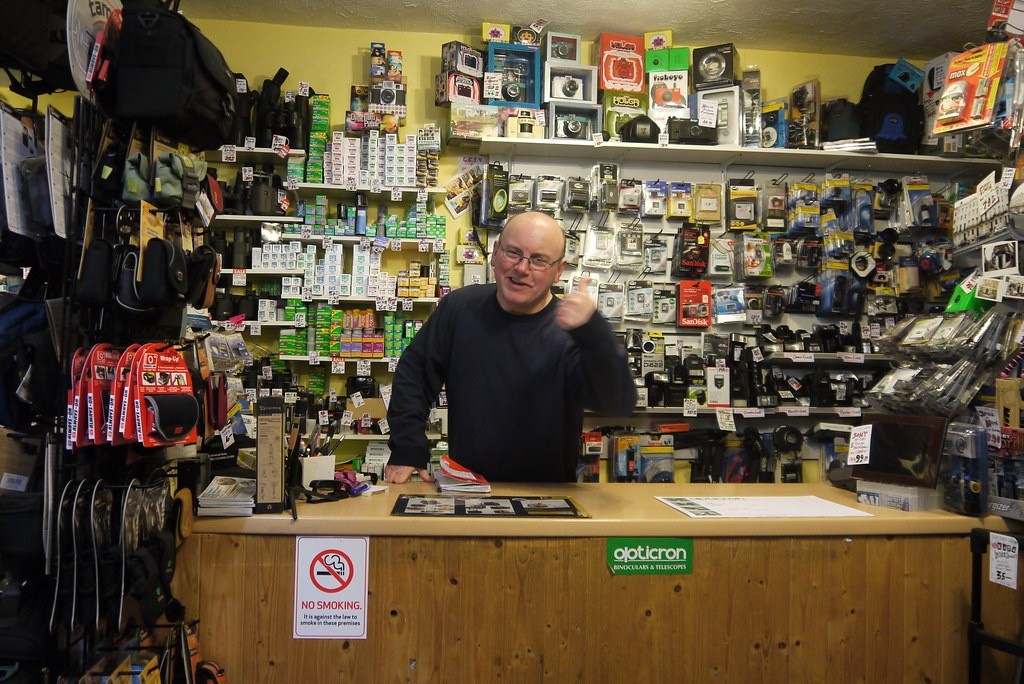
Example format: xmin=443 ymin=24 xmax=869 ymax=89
xmin=434 ymin=454 xmax=491 ymax=492
xmin=198 ymin=476 xmax=257 ymax=517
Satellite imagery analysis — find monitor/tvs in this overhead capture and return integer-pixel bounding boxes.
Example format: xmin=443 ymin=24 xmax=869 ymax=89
xmin=851 ymin=413 xmax=950 ymax=488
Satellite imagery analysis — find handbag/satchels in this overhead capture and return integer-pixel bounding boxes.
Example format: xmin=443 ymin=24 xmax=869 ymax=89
xmin=71 ymin=234 xmax=180 ymax=318
xmin=188 ymin=246 xmax=222 ymax=309
xmin=88 ymin=138 xmax=224 ymax=221
xmin=96 ymin=0 xmax=242 ymax=151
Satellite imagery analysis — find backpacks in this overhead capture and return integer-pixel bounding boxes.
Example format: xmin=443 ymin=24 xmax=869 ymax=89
xmin=0 ymin=289 xmax=68 ymax=435
xmin=859 ymin=63 xmax=925 ymax=155
xmin=1 ymin=1 xmax=66 ymax=97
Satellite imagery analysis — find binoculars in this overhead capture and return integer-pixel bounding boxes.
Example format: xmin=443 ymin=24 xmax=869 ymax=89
xmin=234 ymin=160 xmax=286 ymax=216
xmin=256 ymin=100 xmax=315 ymax=151
xmin=233 ymin=226 xmax=261 ymax=270
xmin=217 ymin=181 xmax=234 ymax=214
xmin=202 ymin=227 xmax=228 ymax=269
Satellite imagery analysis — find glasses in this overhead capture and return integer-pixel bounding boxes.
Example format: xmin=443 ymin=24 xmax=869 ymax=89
xmin=499 ymin=240 xmax=564 ymax=271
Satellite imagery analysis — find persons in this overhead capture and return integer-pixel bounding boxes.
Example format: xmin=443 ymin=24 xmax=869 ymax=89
xmin=384 ymin=211 xmax=637 ymax=484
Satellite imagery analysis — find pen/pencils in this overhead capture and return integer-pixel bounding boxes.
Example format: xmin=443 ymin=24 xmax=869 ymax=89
xmin=304 ymin=421 xmax=345 ymax=457
